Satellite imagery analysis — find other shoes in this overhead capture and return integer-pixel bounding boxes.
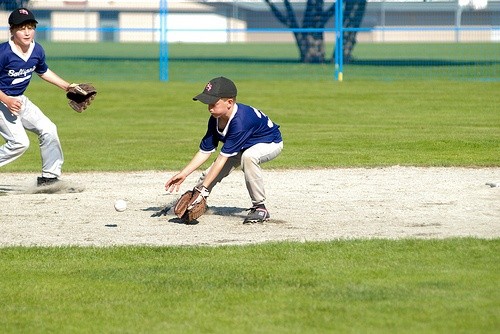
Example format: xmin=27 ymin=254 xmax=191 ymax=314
xmin=37 ymin=177 xmax=59 ymax=191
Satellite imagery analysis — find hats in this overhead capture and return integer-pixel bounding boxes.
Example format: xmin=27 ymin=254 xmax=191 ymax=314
xmin=8 ymin=8 xmax=39 ymax=27
xmin=193 ymin=77 xmax=238 ymax=104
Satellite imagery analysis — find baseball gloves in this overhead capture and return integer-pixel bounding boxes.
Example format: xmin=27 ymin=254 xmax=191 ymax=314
xmin=66 ymin=81 xmax=98 ymax=114
xmin=173 ymin=180 xmax=211 ymax=224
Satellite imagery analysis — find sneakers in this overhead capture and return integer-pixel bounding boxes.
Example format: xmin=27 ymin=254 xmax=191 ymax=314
xmin=243 ymin=206 xmax=270 ymax=224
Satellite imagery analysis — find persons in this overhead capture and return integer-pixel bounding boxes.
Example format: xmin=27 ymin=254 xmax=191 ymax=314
xmin=0 ymin=7 xmax=87 ymax=193
xmin=163 ymin=75 xmax=283 ymax=224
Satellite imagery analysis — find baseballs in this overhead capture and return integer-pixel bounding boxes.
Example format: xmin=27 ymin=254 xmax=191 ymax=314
xmin=114 ymin=199 xmax=127 ymax=212
